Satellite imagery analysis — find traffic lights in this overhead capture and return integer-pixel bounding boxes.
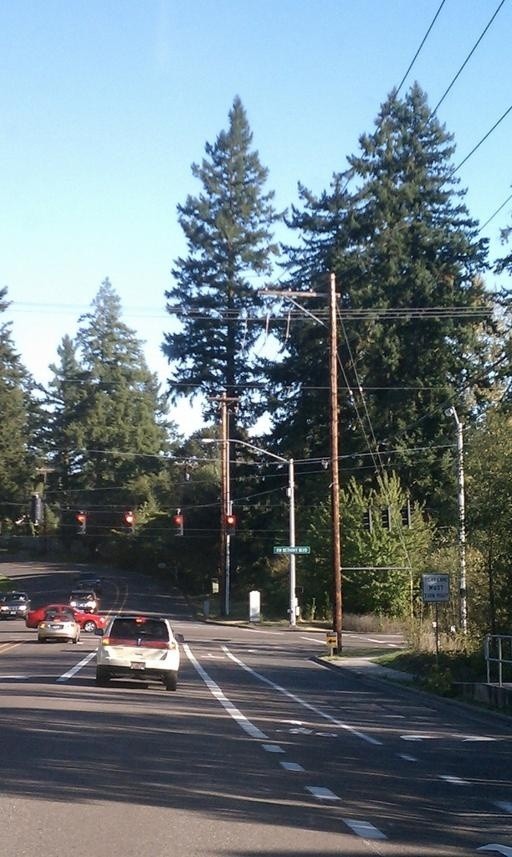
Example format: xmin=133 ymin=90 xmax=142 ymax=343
xmin=76 ymin=514 xmax=87 ymax=535
xmin=126 ymin=512 xmax=135 ymax=534
xmin=174 ymin=514 xmax=185 ymax=537
xmin=360 ymin=503 xmax=414 ymax=532
xmin=226 ymin=515 xmax=236 ymax=536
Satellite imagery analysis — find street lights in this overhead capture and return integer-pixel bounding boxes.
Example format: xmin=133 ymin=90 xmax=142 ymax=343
xmin=202 ymin=437 xmax=297 ymax=628
xmin=444 ymin=404 xmax=465 ymax=639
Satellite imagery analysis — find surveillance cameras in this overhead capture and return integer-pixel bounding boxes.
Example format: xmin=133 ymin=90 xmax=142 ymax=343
xmin=184 ymin=473 xmax=190 ymax=481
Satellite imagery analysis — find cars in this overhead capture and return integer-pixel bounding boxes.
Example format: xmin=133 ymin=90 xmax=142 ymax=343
xmin=0 ymin=563 xmax=106 ymax=644
xmin=95 ymin=616 xmax=184 ymax=690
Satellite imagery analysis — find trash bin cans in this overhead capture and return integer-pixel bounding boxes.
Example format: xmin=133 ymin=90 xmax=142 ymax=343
xmin=208 ymin=592 xmax=225 ymax=619
